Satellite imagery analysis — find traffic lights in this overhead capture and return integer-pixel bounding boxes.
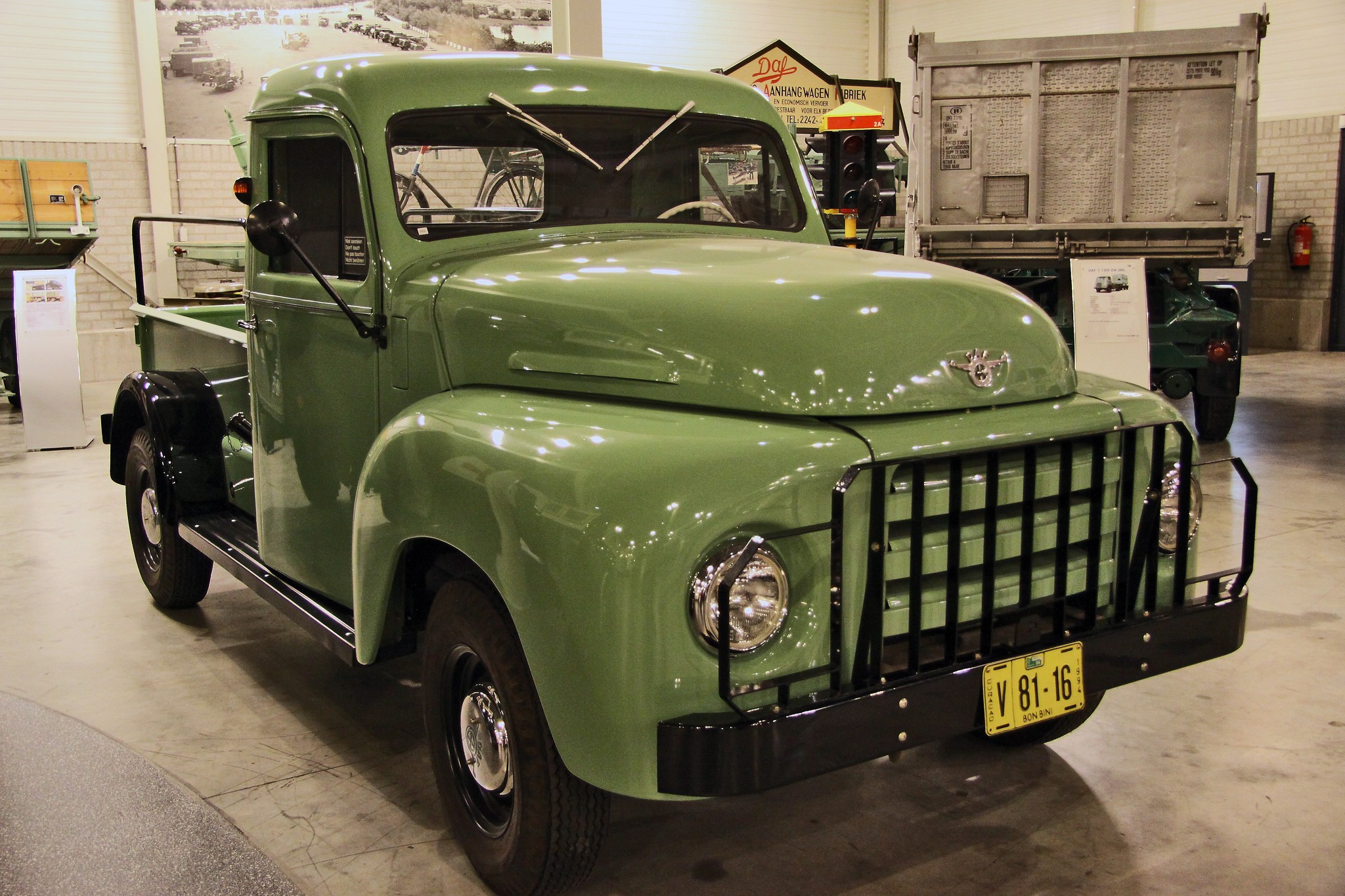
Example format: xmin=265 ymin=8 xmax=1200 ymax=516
xmin=803 ymin=134 xmax=896 ymax=219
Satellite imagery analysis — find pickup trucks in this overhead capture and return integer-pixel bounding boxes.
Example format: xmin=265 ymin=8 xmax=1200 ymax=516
xmin=99 ymin=49 xmax=1257 ymax=896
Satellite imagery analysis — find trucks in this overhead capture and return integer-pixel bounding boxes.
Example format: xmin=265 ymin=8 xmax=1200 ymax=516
xmin=169 ymin=37 xmax=242 ymax=91
xmin=374 ymin=9 xmax=384 ymax=17
xmin=264 ymin=9 xmax=282 ymax=23
xmin=300 ymin=14 xmax=309 ymax=25
xmin=282 ymin=31 xmax=309 ymax=50
xmin=909 ymin=12 xmax=1259 ymax=442
xmin=318 ymin=18 xmax=329 ymax=26
xmin=334 ymin=20 xmax=348 ymax=29
xmin=175 ymin=12 xmax=248 ymax=36
xmin=283 ymin=15 xmax=294 ymax=24
xmin=347 ymin=13 xmax=362 ymax=20
xmin=245 ymin=9 xmax=261 ymax=24
xmin=349 ymin=24 xmax=363 ymax=32
xmin=360 ymin=24 xmax=427 ymax=51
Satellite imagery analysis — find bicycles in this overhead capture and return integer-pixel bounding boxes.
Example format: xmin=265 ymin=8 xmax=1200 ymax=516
xmin=392 ymin=145 xmax=545 ymax=224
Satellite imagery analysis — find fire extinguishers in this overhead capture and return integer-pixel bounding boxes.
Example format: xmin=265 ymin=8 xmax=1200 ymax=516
xmin=1286 ymin=216 xmax=1315 ymax=271
